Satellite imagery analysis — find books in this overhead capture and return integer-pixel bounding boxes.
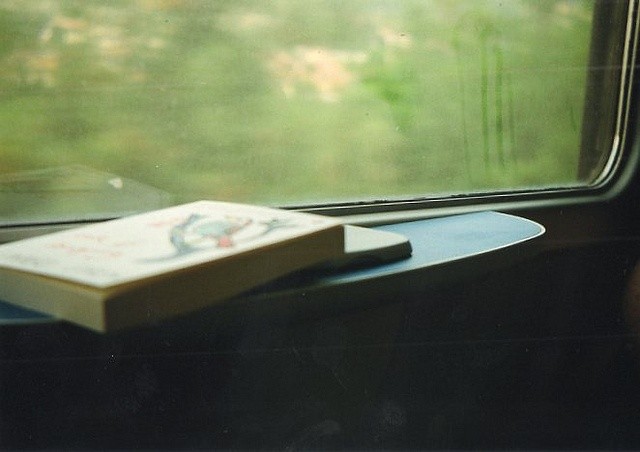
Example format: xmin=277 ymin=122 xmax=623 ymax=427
xmin=0 ymin=197 xmax=346 ymax=336
